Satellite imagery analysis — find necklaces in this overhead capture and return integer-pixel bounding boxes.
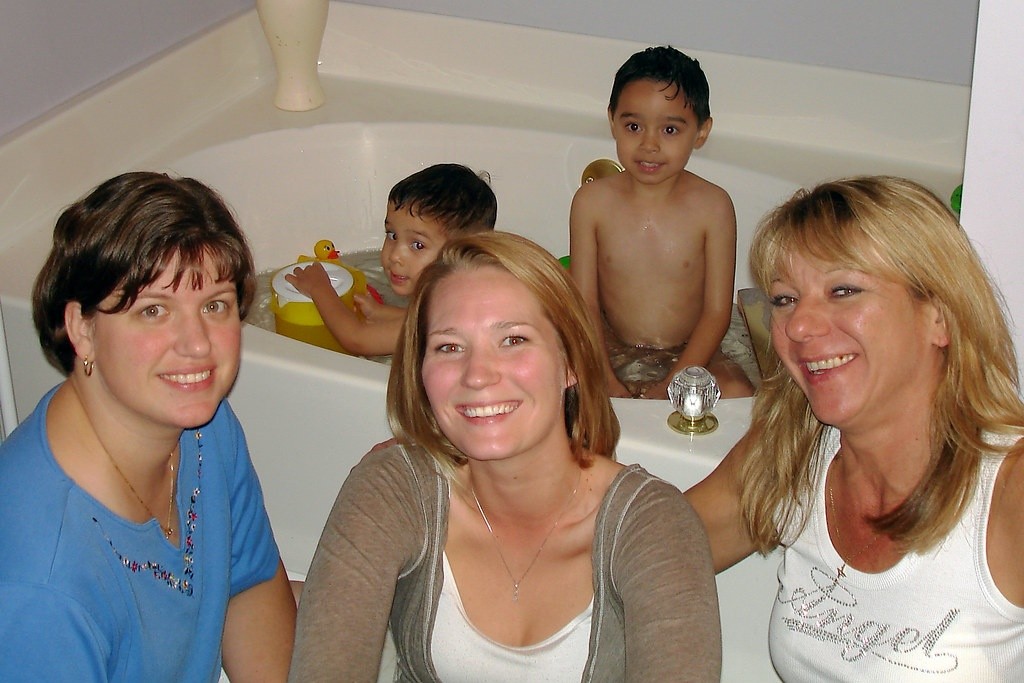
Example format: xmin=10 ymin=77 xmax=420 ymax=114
xmin=75 ymin=387 xmax=177 ymax=539
xmin=471 ymin=469 xmax=582 ymax=600
xmin=828 ymin=452 xmax=882 ymax=583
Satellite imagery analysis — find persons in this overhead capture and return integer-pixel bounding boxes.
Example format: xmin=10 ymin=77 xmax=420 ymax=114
xmin=290 ymin=230 xmax=723 ymax=683
xmin=0 ymin=172 xmax=297 ymax=683
xmin=569 ymin=46 xmax=737 ymax=396
xmin=284 ymin=163 xmax=497 ymax=360
xmin=681 ymin=175 xmax=1024 ymax=683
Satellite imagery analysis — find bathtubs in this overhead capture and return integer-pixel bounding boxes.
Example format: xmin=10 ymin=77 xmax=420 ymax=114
xmin=1 ymin=76 xmax=964 ymax=683
